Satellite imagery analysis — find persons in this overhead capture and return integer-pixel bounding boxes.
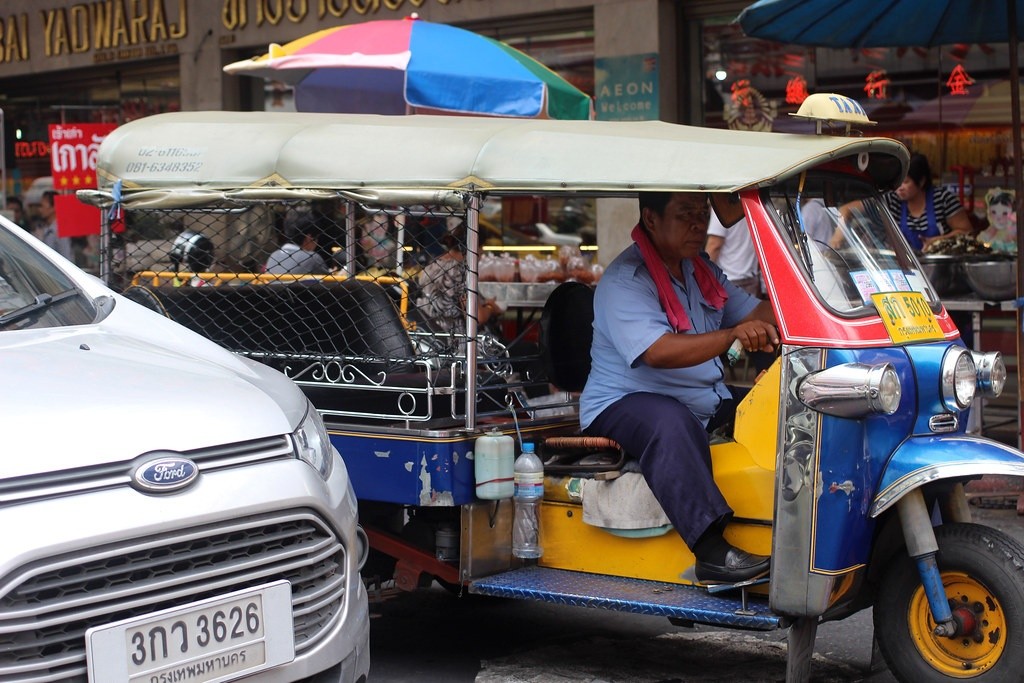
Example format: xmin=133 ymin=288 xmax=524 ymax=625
xmin=706 ymin=200 xmax=839 ymax=297
xmin=579 ymin=193 xmax=780 ymax=584
xmin=413 ymin=224 xmax=502 ymax=360
xmin=0 ymin=190 xmax=72 ymax=264
xmin=264 ymin=219 xmax=328 ymax=275
xmin=827 ymin=150 xmax=976 ymax=297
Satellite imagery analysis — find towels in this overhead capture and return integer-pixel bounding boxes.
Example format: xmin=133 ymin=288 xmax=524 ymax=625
xmin=631 ymin=223 xmax=730 ymax=333
xmin=581 ymin=472 xmax=674 ymax=538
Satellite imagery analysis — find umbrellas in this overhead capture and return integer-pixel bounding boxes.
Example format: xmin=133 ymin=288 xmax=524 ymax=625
xmin=223 ymin=14 xmax=595 ymax=121
xmin=731 ymin=0 xmax=1024 ymax=270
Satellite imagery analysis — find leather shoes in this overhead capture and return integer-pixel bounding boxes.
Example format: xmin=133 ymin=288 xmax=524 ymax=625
xmin=695 ymin=547 xmax=772 ymax=585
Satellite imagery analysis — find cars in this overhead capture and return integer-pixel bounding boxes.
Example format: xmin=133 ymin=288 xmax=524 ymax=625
xmin=0 ymin=214 xmax=373 ymax=682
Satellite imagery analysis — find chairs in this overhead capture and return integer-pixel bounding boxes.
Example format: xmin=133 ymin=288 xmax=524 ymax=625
xmin=538 ymin=281 xmax=596 ymax=512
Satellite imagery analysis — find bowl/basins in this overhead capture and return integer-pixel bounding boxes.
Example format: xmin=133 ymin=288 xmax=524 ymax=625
xmin=918 ymin=256 xmax=964 ymax=296
xmin=964 ymin=262 xmax=1018 ymax=300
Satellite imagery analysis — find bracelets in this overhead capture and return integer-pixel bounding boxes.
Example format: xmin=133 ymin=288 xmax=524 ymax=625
xmin=482 ymin=304 xmax=495 ymax=314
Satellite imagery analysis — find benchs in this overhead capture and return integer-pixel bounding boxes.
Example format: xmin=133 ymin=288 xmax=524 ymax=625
xmin=119 ymin=278 xmax=510 ymax=428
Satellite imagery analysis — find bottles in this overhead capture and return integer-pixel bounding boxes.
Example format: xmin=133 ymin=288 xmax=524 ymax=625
xmin=475 ymin=430 xmax=514 ymax=499
xmin=478 ymin=251 xmax=602 ymax=282
xmin=513 ymin=443 xmax=545 ymax=558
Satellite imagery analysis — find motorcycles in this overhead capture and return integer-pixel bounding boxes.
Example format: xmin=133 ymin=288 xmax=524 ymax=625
xmin=97 ymin=110 xmax=1024 ymax=682
